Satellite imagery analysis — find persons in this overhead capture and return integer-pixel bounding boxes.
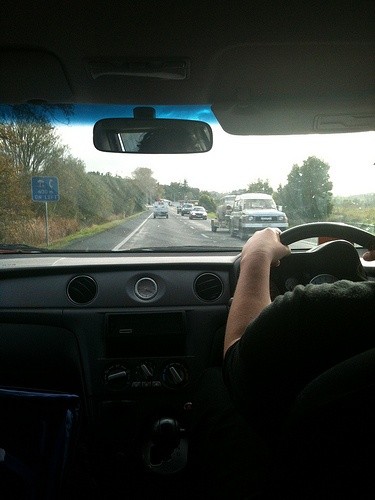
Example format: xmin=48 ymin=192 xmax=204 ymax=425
xmin=222 ymin=225 xmax=375 ymax=499
xmin=136 ymin=127 xmax=201 ymax=151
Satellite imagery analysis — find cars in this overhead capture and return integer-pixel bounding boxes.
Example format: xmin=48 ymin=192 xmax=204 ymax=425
xmin=189 ymin=206 xmax=208 ymax=219
xmin=153 ymin=198 xmax=193 ymax=218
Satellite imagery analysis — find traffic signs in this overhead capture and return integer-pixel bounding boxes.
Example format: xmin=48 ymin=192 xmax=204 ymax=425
xmin=31 ymin=175 xmax=60 ymax=203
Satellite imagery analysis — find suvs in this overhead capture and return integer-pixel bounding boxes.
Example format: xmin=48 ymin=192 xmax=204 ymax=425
xmin=210 ymin=194 xmax=242 ymax=234
xmin=229 ymin=192 xmax=288 ymax=241
